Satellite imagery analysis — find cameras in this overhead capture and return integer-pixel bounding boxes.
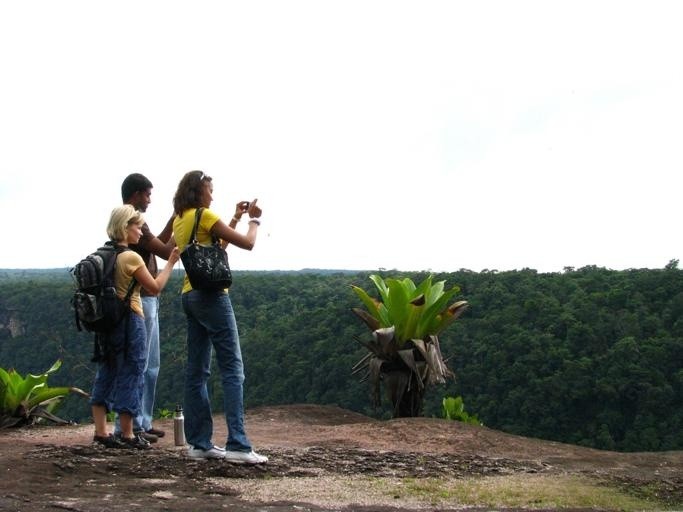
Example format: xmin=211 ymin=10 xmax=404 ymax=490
xmin=244 ymin=202 xmax=251 ymax=208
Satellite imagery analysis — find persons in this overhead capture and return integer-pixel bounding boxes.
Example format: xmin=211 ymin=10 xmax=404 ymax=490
xmin=89 ymin=173 xmax=180 ymax=449
xmin=173 ymin=169 xmax=269 ymax=464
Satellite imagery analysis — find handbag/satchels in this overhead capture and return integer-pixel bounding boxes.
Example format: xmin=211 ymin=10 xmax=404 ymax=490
xmin=180 ymin=206 xmax=232 ymax=290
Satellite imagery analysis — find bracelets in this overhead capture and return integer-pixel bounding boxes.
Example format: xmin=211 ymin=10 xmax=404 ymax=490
xmin=232 ymin=216 xmax=240 ymax=222
xmin=248 ymin=218 xmax=261 ymax=225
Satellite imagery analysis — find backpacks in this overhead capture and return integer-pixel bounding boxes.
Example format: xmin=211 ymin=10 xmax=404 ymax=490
xmin=70 ymin=241 xmax=138 ymax=332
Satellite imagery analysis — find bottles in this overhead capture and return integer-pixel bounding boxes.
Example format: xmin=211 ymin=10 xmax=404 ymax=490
xmin=173 ymin=404 xmax=186 ymax=447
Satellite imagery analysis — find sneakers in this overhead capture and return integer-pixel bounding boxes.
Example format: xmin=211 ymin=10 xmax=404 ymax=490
xmin=94 ymin=429 xmax=164 ymax=448
xmin=187 ymin=445 xmax=226 ymax=460
xmin=226 ymin=450 xmax=269 ymax=464
